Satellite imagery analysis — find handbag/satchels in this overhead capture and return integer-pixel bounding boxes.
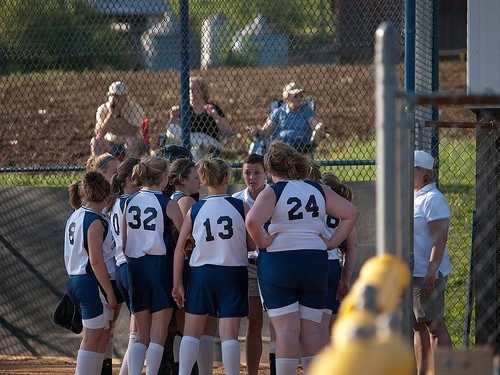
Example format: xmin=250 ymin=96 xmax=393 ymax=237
xmin=52 ymin=290 xmax=83 ymax=335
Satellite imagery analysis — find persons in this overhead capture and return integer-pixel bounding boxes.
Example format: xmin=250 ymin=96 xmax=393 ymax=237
xmin=63 ymin=141 xmax=359 ymax=375
xmin=90 ymin=81 xmax=146 ymax=160
xmin=166 ymin=76 xmax=227 ymax=163
xmin=249 ymin=82 xmax=325 ymax=156
xmin=413 ymin=150 xmax=452 ymax=375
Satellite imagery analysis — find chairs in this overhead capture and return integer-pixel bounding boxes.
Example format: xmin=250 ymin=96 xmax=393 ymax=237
xmin=248 ymin=100 xmax=318 ymax=159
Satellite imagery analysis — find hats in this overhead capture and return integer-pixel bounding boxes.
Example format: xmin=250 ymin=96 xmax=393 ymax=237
xmin=414 ymin=151 xmax=435 ymax=170
xmin=283 ymin=83 xmax=305 ymax=98
xmin=107 ymin=81 xmax=129 ymax=95
